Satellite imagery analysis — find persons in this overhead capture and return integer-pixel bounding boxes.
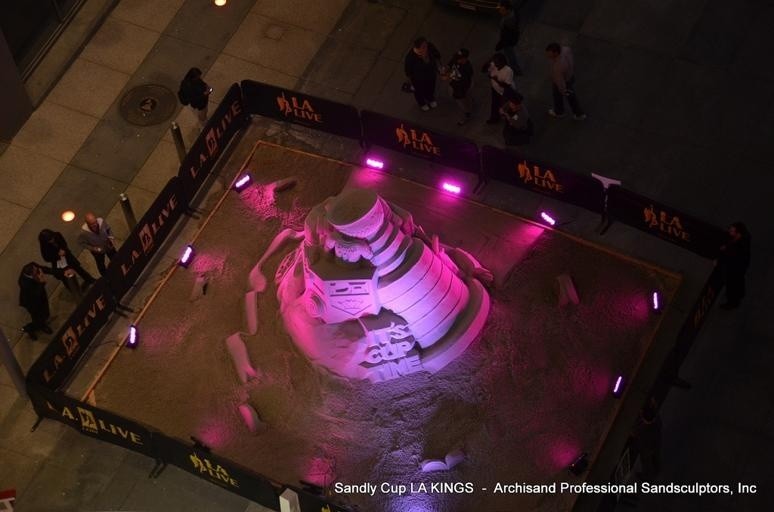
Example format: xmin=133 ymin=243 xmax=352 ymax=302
xmin=544 ymin=42 xmax=585 ymax=121
xmin=629 ymin=398 xmax=663 ymax=447
xmin=17 ymin=260 xmax=74 ymax=340
xmin=479 ymin=1 xmax=524 ymax=77
xmin=406 ymin=35 xmax=442 ymax=112
xmin=38 ymin=228 xmax=96 ymax=293
xmin=713 ymin=219 xmax=751 ymax=308
xmin=183 ymin=68 xmax=212 ymax=132
xmin=443 ymin=47 xmax=478 ymax=126
xmin=486 ymin=49 xmax=515 ymax=123
xmin=76 ymin=212 xmax=117 ymax=276
xmin=500 ymin=88 xmax=535 ymax=145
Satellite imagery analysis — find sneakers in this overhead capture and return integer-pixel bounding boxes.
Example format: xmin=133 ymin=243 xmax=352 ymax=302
xmin=418 ymin=100 xmax=588 ymax=128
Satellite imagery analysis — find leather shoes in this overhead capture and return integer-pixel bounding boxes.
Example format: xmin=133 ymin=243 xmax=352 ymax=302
xmin=18 ymin=324 xmax=52 ymax=341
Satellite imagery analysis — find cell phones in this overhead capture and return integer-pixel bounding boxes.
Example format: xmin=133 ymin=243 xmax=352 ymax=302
xmin=208 ymin=88 xmax=213 ymax=92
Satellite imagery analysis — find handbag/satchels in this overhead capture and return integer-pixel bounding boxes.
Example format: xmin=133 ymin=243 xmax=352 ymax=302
xmin=501 ymin=126 xmax=530 ymax=147
